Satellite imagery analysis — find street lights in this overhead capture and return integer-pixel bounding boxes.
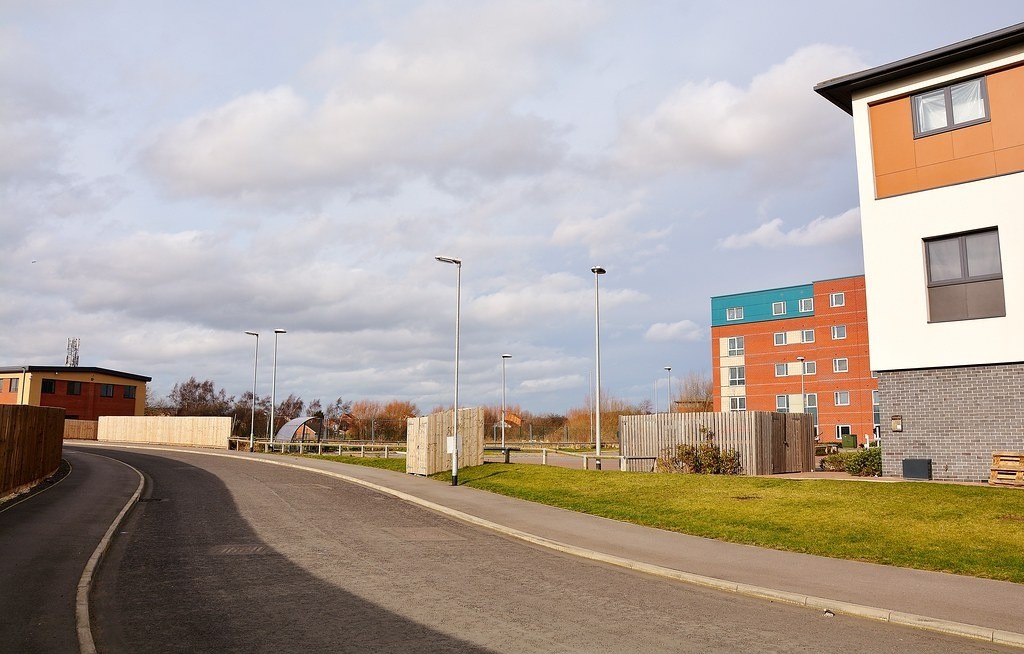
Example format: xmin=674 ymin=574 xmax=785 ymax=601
xmin=435 ymin=255 xmax=462 ymax=486
xmin=664 ymin=366 xmax=672 ymax=414
xmin=797 ymin=357 xmax=805 ymax=413
xmin=502 ymin=354 xmax=512 ymax=454
xmin=270 ymin=328 xmax=287 ymax=452
xmin=590 ymin=265 xmax=606 ymax=470
xmin=245 ymin=331 xmax=258 ymax=452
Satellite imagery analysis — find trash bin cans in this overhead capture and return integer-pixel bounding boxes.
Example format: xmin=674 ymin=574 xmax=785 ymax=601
xmin=842 ymin=434 xmax=857 ymax=448
